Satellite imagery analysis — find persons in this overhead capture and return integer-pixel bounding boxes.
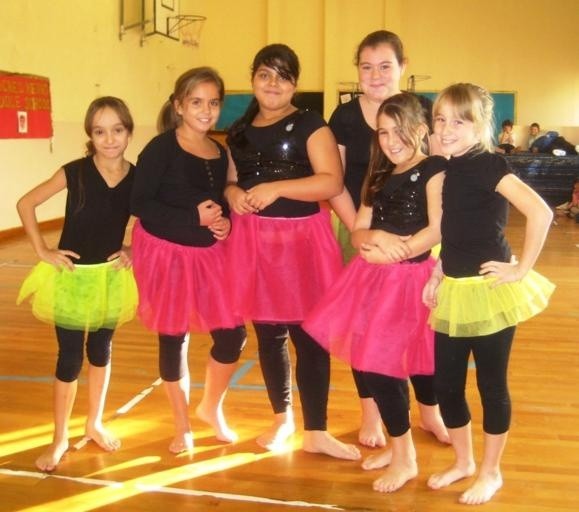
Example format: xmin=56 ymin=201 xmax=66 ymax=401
xmin=326 ymin=27 xmax=456 ymax=449
xmin=222 ymin=41 xmax=362 ymax=463
xmin=566 ymin=178 xmax=579 ymax=219
xmin=415 ymin=79 xmax=558 ymax=507
xmin=16 ymin=94 xmax=138 ymax=473
xmin=526 ymin=120 xmax=578 ymax=158
xmin=127 ymin=64 xmax=250 ymax=454
xmin=347 ymin=88 xmax=448 ymax=497
xmin=492 ymin=117 xmax=522 ymax=157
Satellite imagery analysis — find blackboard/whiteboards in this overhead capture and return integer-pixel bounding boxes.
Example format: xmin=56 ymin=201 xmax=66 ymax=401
xmin=212 ymin=92 xmax=325 ymax=131
xmin=338 ymin=92 xmax=516 ymax=146
